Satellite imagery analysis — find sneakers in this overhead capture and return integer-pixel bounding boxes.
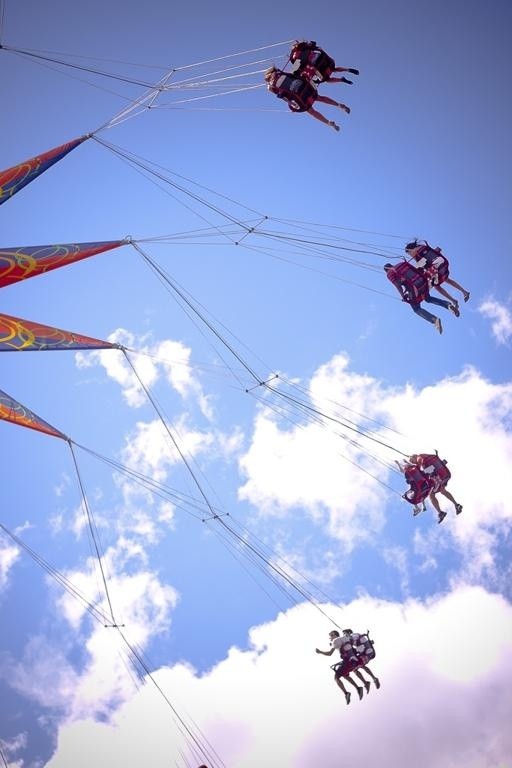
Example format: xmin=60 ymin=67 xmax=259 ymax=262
xmin=435 ymin=291 xmax=471 ymax=335
xmin=438 ymin=502 xmax=462 ymax=523
xmin=339 ymin=67 xmax=360 ymax=86
xmin=412 ymin=505 xmax=427 ymax=516
xmin=326 ymin=103 xmax=350 ymax=132
xmin=346 ymin=677 xmax=380 ymax=704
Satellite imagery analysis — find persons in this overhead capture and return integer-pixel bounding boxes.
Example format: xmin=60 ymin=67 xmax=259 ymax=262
xmin=342 ymin=628 xmax=381 ymax=694
xmin=287 ymin=40 xmax=360 ymax=85
xmin=394 ymin=459 xmax=433 ymax=516
xmin=315 ymin=630 xmax=363 ymax=705
xmin=264 ymin=66 xmax=351 ymax=132
xmin=410 ymin=454 xmax=463 ymax=525
xmin=405 ymin=242 xmax=470 ymax=311
xmin=384 ymin=263 xmax=460 ymax=335
